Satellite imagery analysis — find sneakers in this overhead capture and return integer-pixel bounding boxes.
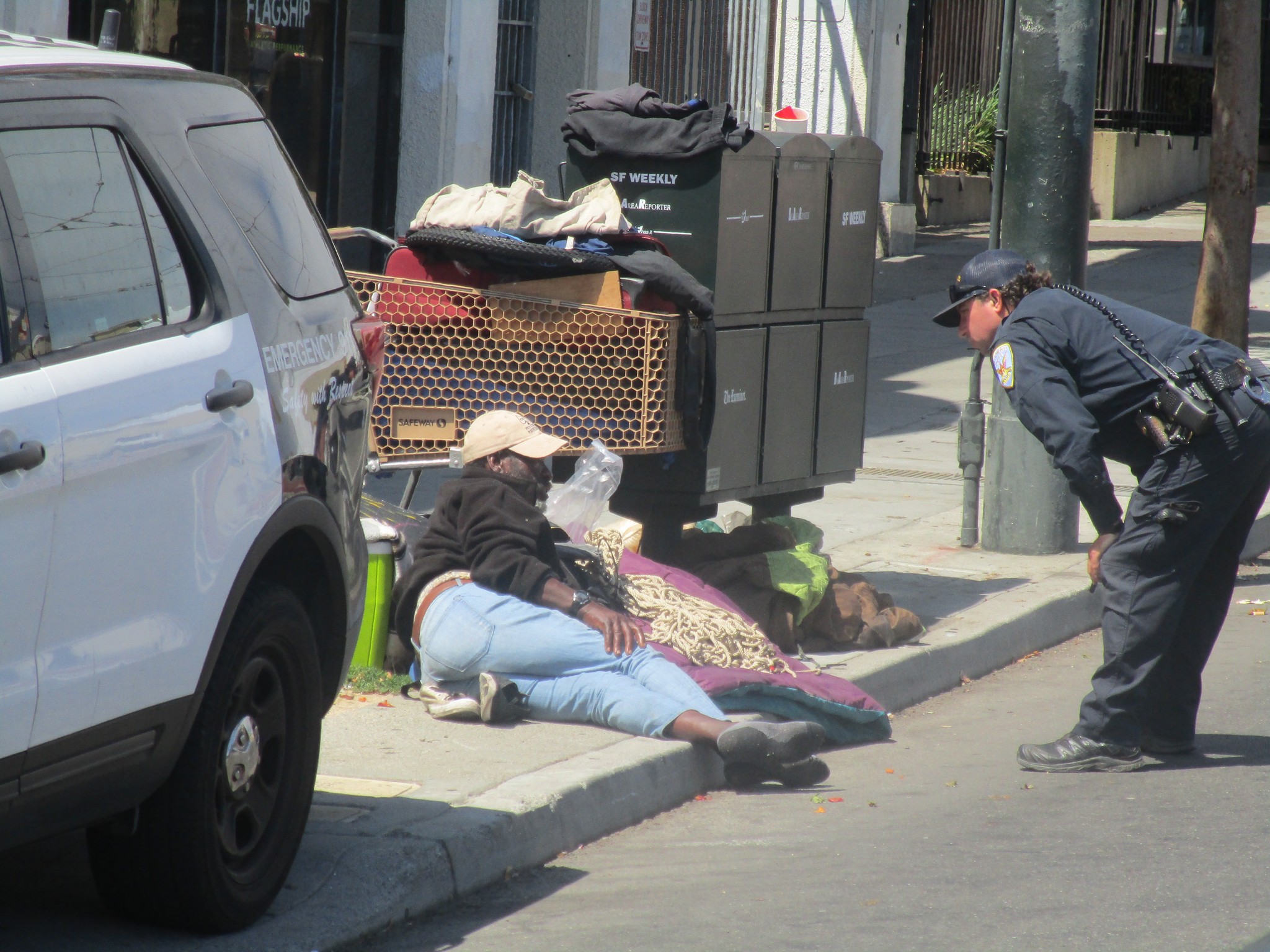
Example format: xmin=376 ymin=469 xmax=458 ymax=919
xmin=1141 ymin=731 xmax=1199 ymax=753
xmin=477 ymin=669 xmax=531 ymax=724
xmin=418 ymin=683 xmax=480 ymax=719
xmin=1016 ymin=731 xmax=1147 ymax=773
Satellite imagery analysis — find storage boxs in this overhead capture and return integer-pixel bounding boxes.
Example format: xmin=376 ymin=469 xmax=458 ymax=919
xmin=487 ymin=271 xmax=625 ymax=343
xmin=349 ymin=537 xmax=394 ymax=668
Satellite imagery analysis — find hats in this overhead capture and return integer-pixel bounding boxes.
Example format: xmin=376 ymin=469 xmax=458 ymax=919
xmin=461 ymin=410 xmax=569 ymax=466
xmin=933 ymin=249 xmax=1030 ymax=328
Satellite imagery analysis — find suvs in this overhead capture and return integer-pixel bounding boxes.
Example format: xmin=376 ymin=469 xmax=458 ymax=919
xmin=3 ymin=30 xmax=388 ymax=937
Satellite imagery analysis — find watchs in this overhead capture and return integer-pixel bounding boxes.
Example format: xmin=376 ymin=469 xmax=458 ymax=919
xmin=569 ymin=589 xmax=592 ymax=618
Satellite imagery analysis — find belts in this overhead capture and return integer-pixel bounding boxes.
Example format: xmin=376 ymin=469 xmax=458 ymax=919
xmin=412 ymin=579 xmax=475 ymax=667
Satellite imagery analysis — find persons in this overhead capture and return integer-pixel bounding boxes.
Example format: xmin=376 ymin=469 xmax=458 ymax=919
xmin=388 ymin=411 xmax=831 ymax=790
xmin=932 ymin=248 xmax=1270 ymax=773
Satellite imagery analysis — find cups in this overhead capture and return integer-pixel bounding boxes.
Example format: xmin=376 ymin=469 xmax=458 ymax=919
xmin=773 ymin=107 xmax=809 ymax=133
